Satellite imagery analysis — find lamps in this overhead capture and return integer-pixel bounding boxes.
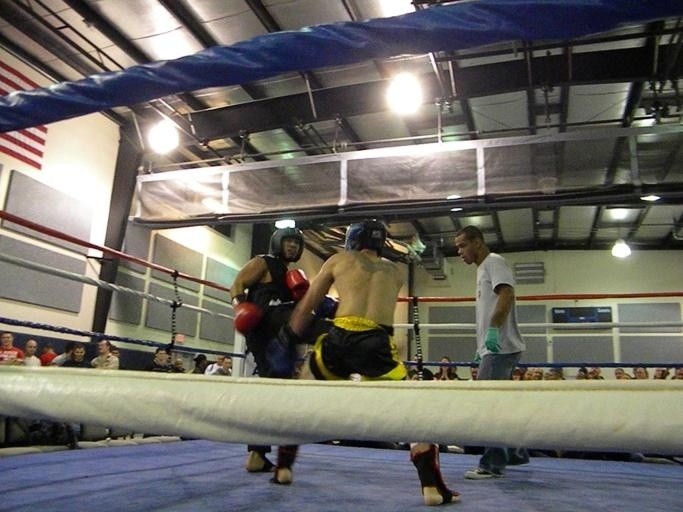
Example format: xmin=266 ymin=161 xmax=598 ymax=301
xmin=612 ymin=219 xmax=631 ymax=258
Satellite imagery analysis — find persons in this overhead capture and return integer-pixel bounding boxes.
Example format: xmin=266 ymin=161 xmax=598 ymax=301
xmin=511 ymin=366 xmax=683 ymax=463
xmin=470 ymin=367 xmax=478 ymax=380
xmin=0 ymin=332 xmax=232 ymax=447
xmin=267 ymin=219 xmax=462 ymax=507
xmin=432 ymin=356 xmax=460 ymax=380
xmin=408 ymin=353 xmax=433 ymax=380
xmin=230 ymin=226 xmax=333 ymax=474
xmin=455 ymin=224 xmax=530 ymax=481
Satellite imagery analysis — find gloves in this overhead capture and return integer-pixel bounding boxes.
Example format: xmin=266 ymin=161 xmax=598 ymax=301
xmin=263 ymin=321 xmax=304 ymax=372
xmin=230 ymin=293 xmax=261 ymax=333
xmin=315 ymin=297 xmax=341 ymax=320
xmin=484 ymin=327 xmax=503 ymax=354
xmin=286 ymin=269 xmax=309 ymax=302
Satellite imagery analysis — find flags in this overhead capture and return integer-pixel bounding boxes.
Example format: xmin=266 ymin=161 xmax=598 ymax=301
xmin=0 ymin=51 xmax=55 ymax=170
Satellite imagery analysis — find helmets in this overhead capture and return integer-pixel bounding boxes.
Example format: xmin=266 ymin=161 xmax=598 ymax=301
xmin=269 ymin=227 xmax=304 ymax=264
xmin=345 ymin=218 xmax=387 ymax=252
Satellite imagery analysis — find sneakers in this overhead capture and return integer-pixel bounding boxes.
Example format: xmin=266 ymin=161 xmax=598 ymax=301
xmin=464 ymin=467 xmax=501 ymax=480
